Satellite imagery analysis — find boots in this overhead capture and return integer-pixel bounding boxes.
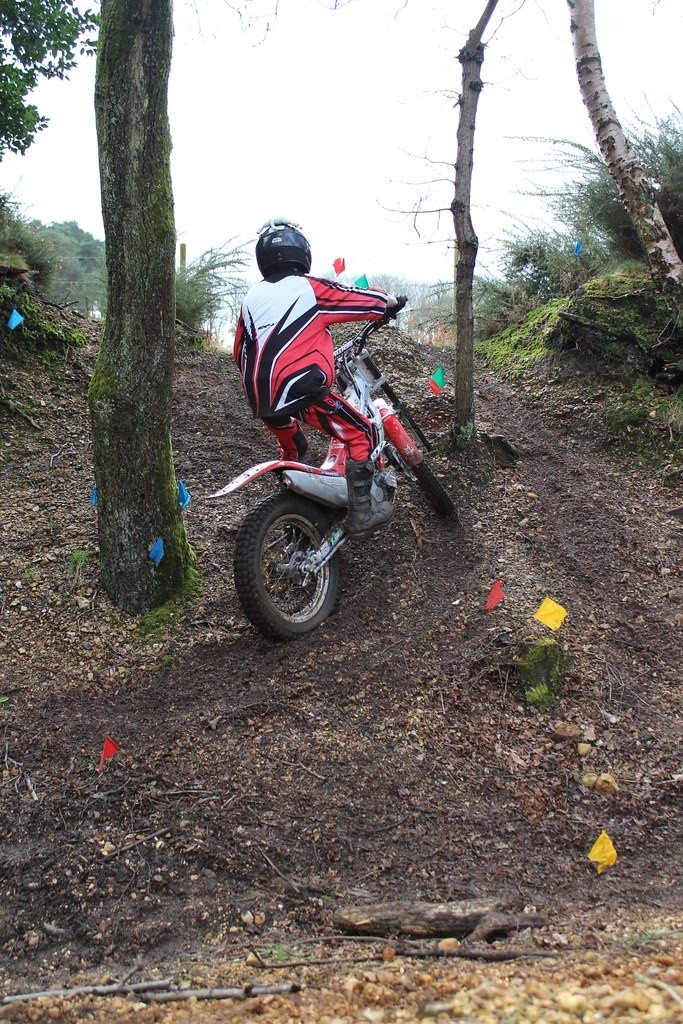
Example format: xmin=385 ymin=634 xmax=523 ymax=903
xmin=345 ymin=459 xmax=396 ymax=538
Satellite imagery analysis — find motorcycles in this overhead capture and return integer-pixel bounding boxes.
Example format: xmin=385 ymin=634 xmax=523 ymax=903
xmin=201 ymin=298 xmax=454 ymax=642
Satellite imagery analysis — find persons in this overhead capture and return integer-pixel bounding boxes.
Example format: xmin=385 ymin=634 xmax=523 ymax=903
xmin=232 ymin=217 xmax=398 ymax=546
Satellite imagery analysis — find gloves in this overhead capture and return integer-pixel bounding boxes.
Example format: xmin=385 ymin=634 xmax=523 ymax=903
xmin=385 ymin=297 xmax=406 ymax=319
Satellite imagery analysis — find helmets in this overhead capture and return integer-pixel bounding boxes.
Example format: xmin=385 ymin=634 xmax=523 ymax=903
xmin=255 ymin=224 xmax=312 ymax=278
